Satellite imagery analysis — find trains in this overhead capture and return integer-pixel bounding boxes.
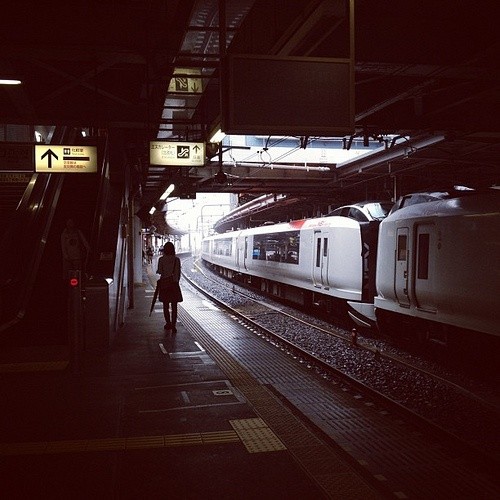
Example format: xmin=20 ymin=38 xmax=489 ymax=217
xmin=201 ymin=185 xmax=500 ymax=362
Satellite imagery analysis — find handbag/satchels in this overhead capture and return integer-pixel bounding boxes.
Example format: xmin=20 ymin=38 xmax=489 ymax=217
xmin=156 ymin=275 xmax=174 ymax=292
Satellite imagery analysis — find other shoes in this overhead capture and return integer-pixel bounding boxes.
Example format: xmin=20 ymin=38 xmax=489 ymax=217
xmin=163 ymin=324 xmax=177 ymax=332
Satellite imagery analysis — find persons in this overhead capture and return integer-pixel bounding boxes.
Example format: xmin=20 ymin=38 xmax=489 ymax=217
xmin=61 ymin=216 xmax=86 ymax=286
xmin=156 ymin=241 xmax=183 ymax=330
